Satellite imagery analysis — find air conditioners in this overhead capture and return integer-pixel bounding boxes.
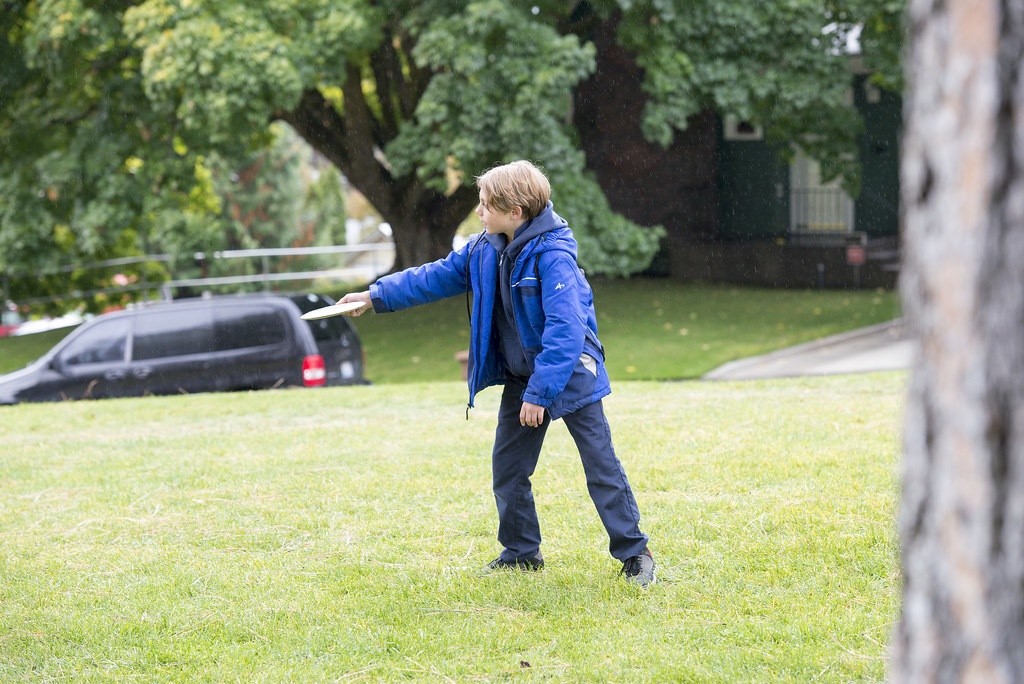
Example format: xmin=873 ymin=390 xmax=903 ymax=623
xmin=721 ymin=112 xmax=763 ymax=141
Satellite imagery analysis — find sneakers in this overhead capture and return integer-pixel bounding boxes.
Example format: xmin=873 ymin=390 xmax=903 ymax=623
xmin=617 ymin=547 xmax=656 ymax=591
xmin=478 ymin=551 xmax=544 ymax=575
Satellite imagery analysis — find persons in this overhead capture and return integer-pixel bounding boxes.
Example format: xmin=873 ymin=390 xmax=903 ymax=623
xmin=334 ymin=160 xmax=657 ymax=591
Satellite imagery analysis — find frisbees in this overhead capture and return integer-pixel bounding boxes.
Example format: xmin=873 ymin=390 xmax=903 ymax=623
xmin=300 ymin=301 xmax=367 ymax=321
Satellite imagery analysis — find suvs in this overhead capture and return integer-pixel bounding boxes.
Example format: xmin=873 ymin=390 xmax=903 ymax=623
xmin=0 ymin=289 xmax=376 ymax=411
